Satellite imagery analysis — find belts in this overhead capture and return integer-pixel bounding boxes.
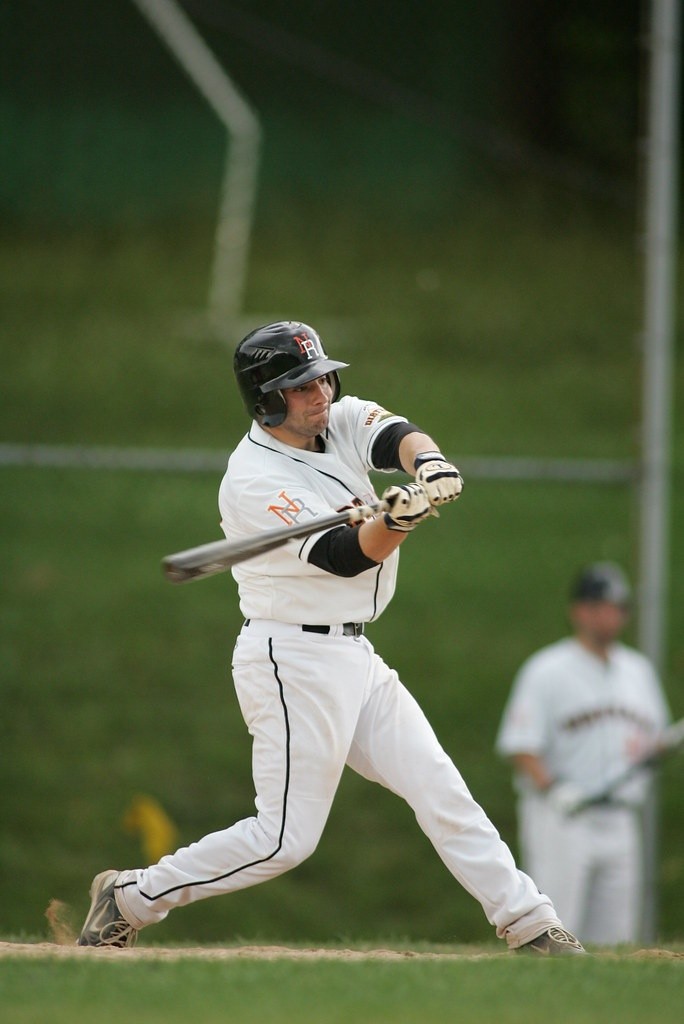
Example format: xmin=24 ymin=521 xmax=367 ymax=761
xmin=244 ymin=618 xmax=365 ymax=637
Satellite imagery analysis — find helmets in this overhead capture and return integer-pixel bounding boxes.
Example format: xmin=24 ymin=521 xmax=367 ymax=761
xmin=566 ymin=562 xmax=632 ymax=610
xmin=233 ymin=321 xmax=350 ymax=428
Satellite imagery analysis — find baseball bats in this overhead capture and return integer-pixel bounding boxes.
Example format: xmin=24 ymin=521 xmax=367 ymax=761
xmin=162 ymin=473 xmax=463 ymax=585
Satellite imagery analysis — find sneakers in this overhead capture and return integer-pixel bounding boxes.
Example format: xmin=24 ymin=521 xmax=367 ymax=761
xmin=78 ymin=870 xmax=138 ymax=948
xmin=514 ymin=926 xmax=587 ymax=958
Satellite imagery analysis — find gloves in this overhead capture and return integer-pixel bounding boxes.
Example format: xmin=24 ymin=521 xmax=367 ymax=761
xmin=382 ymin=482 xmax=431 ymax=533
xmin=542 ymin=772 xmax=597 ymax=818
xmin=413 ymin=451 xmax=465 ymax=509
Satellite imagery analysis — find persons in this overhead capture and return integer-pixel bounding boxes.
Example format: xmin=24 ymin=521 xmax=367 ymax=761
xmin=495 ymin=562 xmax=676 ymax=946
xmin=76 ymin=319 xmax=586 ymax=958
